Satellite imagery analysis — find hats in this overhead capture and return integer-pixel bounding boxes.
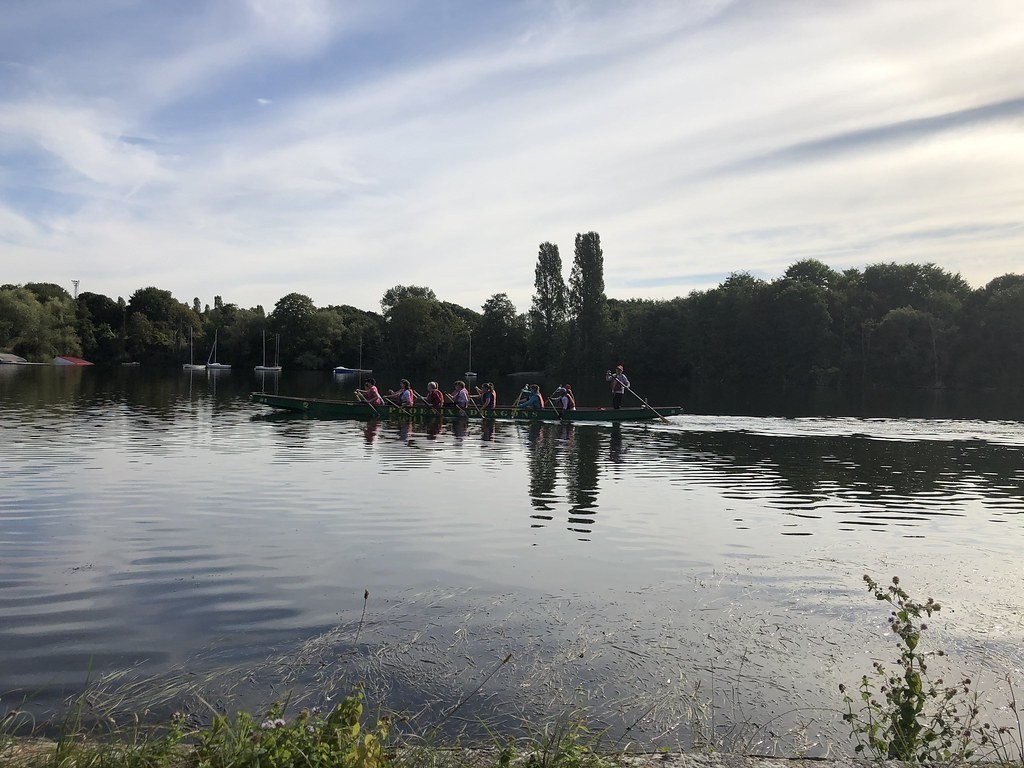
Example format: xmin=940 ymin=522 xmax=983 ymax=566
xmin=564 ymin=384 xmax=571 ymax=389
xmin=617 ymin=365 xmax=623 ymax=371
xmin=556 ymin=388 xmax=566 ymax=392
xmin=364 ymin=378 xmax=375 ymax=383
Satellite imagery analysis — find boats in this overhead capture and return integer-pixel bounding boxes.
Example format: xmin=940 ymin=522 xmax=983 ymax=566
xmin=250 ymin=391 xmax=683 ymax=421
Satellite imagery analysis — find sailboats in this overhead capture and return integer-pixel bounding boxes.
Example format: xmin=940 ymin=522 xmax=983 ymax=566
xmin=182 ymin=326 xmax=206 ymax=372
xmin=253 ymin=330 xmax=282 ymax=372
xmin=206 ymin=327 xmax=233 ymax=369
xmin=333 ymin=335 xmax=373 ymax=374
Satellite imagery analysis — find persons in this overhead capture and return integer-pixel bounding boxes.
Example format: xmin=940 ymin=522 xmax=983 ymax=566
xmin=355 ymin=378 xmax=577 ymax=413
xmin=606 ymin=366 xmax=629 ymax=409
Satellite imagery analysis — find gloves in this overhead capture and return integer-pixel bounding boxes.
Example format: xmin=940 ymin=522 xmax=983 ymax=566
xmin=558 ymin=412 xmax=564 ymax=420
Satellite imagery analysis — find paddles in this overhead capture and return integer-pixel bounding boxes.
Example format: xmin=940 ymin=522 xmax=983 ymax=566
xmin=510 ymin=391 xmax=524 ymax=419
xmin=444 ymin=391 xmax=470 ymax=419
xmin=547 ymin=398 xmax=563 ymax=421
xmin=355 ymin=390 xmax=381 ymax=416
xmin=608 ymin=372 xmax=669 ymax=423
xmin=475 ymin=386 xmax=481 ymax=397
xmin=413 ymin=389 xmax=444 ymax=418
xmin=469 ymin=397 xmax=486 ymax=420
xmin=545 ymin=386 xmax=561 ymax=405
xmin=383 ymin=396 xmax=412 ymax=416
